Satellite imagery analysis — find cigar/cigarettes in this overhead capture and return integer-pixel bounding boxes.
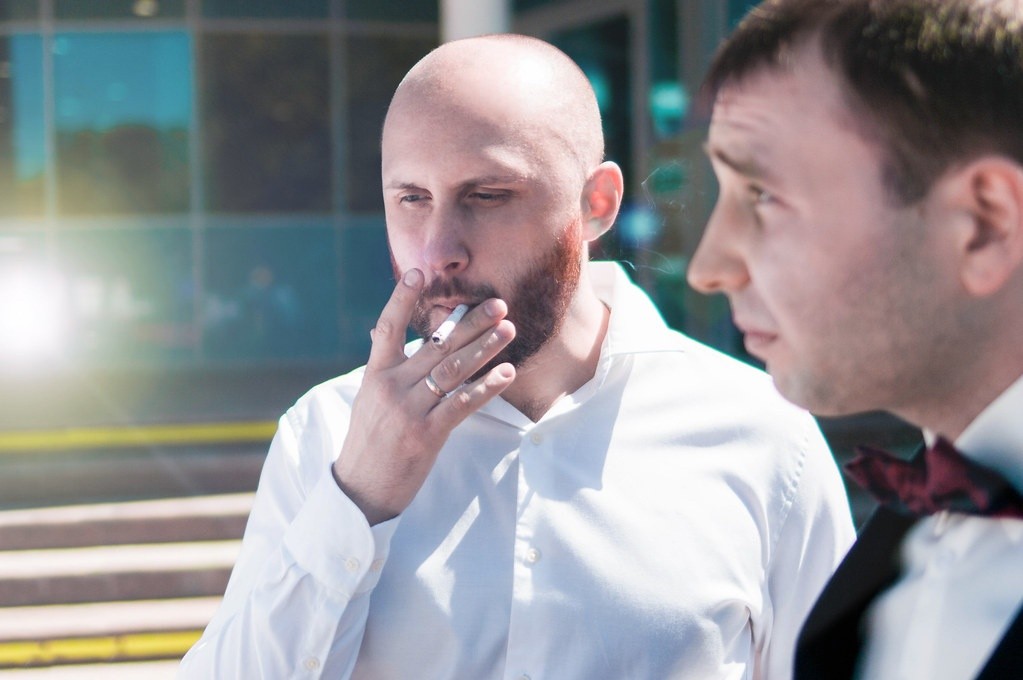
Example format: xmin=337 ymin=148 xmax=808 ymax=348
xmin=432 ymin=304 xmax=469 ymax=345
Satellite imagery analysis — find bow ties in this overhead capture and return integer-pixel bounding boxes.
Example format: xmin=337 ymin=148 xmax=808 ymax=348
xmin=845 ymin=435 xmax=1023 ymax=519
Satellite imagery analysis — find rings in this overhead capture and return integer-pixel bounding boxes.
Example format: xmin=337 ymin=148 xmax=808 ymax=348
xmin=425 ymin=373 xmax=446 ymax=399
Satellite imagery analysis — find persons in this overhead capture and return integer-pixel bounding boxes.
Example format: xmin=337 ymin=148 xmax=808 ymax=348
xmin=171 ymin=34 xmax=857 ymax=680
xmin=688 ymin=0 xmax=1023 ymax=680
